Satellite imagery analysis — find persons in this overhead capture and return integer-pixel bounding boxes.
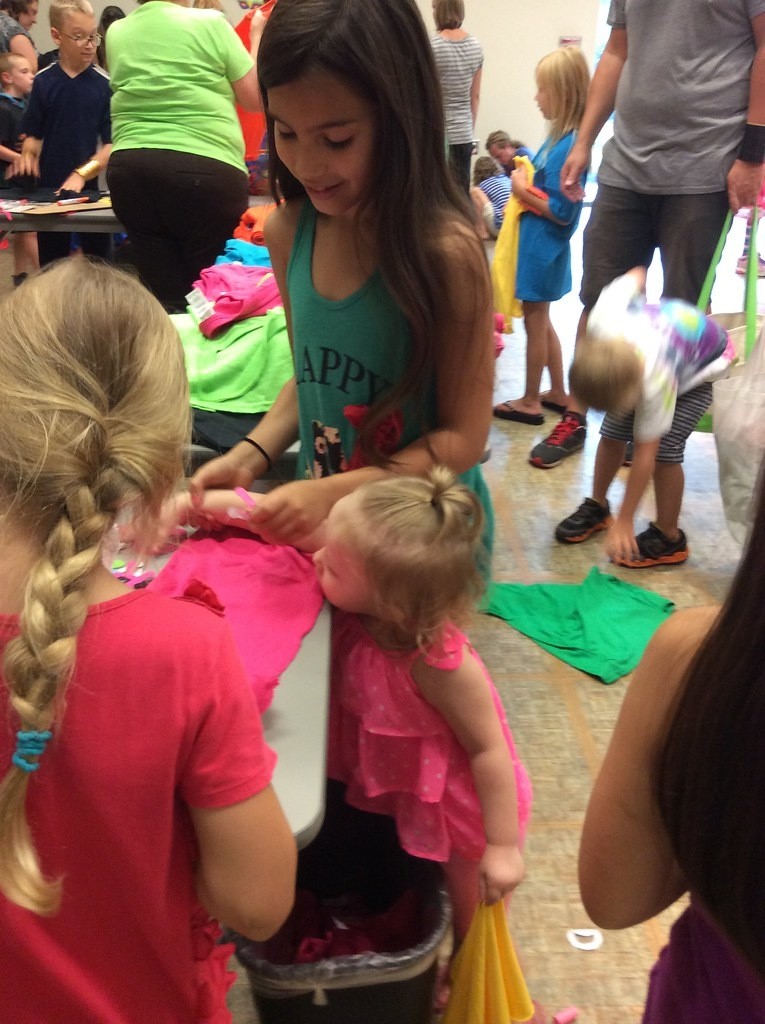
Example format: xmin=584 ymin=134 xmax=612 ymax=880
xmin=0 ymin=0 xmax=269 ymax=304
xmin=490 ymin=43 xmax=592 ymax=424
xmin=577 ymin=447 xmax=765 ymax=1024
xmin=469 ymin=130 xmax=535 ymax=238
xmin=119 ymin=461 xmax=548 ymax=1024
xmin=187 ymin=0 xmax=496 ymax=601
xmin=0 ymin=251 xmax=299 ymax=1024
xmin=428 ymin=0 xmax=484 ymax=221
xmin=555 ymin=265 xmax=740 ymax=569
xmin=529 ymin=0 xmax=765 ymax=470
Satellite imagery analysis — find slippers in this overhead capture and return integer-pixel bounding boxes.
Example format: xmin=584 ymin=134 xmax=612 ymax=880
xmin=540 ymin=390 xmax=567 ymax=413
xmin=494 ymin=400 xmax=544 ymax=425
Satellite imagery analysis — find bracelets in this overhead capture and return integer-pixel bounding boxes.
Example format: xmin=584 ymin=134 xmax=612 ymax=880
xmin=74 ymin=160 xmax=104 ymax=180
xmin=242 ymin=437 xmax=272 ymax=467
xmin=737 ymin=123 xmax=765 ymax=163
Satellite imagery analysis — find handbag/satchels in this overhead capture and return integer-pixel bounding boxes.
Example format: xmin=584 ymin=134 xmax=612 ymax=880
xmin=710 ymin=320 xmax=765 ymax=548
xmin=688 ymin=200 xmax=765 ymax=434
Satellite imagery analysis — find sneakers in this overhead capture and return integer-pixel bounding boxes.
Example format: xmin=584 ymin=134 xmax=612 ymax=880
xmin=528 ymin=412 xmax=587 ymax=467
xmin=620 ymin=522 xmax=687 ymax=568
xmin=623 ymin=442 xmax=634 ymax=465
xmin=556 ymin=497 xmax=613 ymax=541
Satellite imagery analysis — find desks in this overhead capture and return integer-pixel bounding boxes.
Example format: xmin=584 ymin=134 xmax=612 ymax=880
xmin=97 ymin=502 xmax=330 ymax=850
xmin=0 ymin=204 xmax=127 ymax=233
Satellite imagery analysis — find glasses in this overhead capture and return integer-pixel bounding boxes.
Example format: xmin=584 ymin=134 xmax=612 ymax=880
xmin=59 ymin=31 xmax=104 ymax=47
